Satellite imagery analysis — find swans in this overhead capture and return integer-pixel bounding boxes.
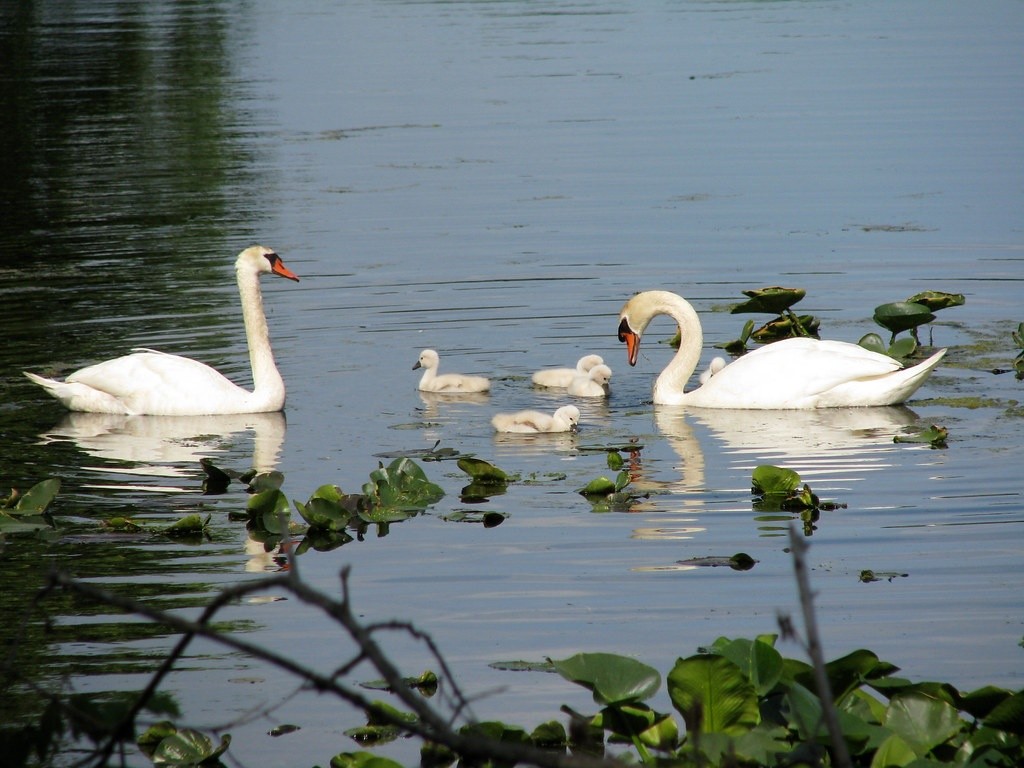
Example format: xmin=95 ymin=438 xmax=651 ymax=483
xmin=618 ymin=290 xmax=950 ymax=409
xmin=411 ymin=348 xmax=611 ymax=434
xmin=24 ymin=244 xmax=300 ymax=415
